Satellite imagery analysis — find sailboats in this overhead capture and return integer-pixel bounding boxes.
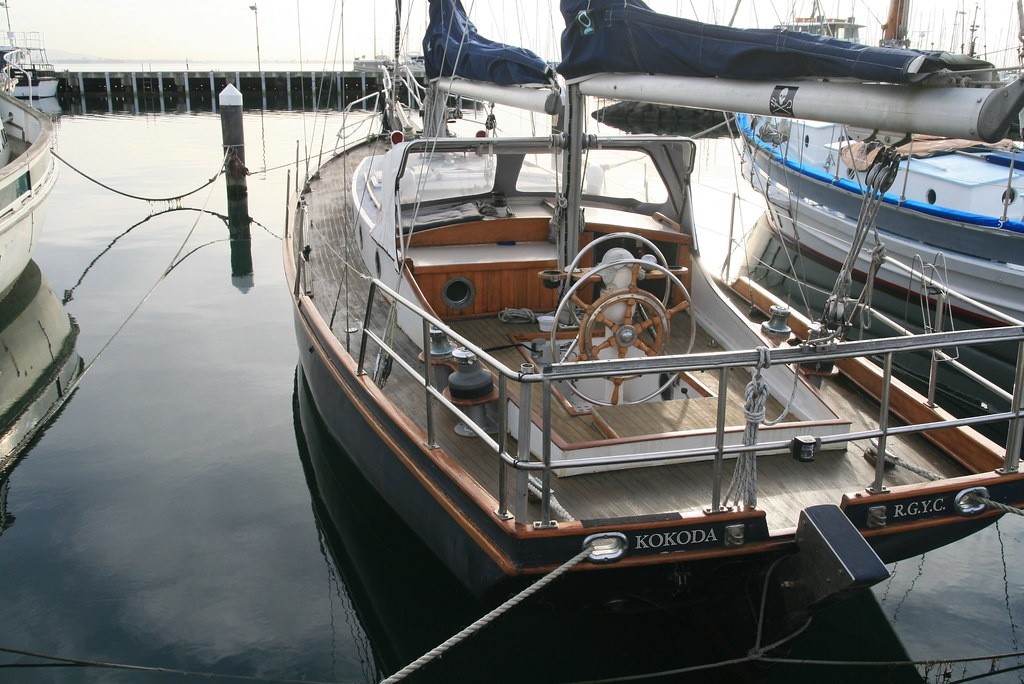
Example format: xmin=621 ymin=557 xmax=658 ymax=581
xmin=289 ymin=350 xmax=930 ymax=683
xmin=723 ymin=0 xmax=1024 ymax=342
xmin=280 ymin=0 xmax=1024 ymax=620
xmin=734 ymin=208 xmax=1024 ymax=462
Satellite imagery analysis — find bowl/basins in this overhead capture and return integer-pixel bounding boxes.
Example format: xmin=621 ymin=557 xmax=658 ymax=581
xmin=537 ymin=316 xmax=554 ymax=332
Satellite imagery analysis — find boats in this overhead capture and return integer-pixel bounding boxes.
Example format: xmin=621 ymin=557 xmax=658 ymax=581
xmin=0 ymin=47 xmax=60 ymax=296
xmin=587 ymin=0 xmax=870 ymax=125
xmin=0 ymin=0 xmax=60 ymax=100
xmin=0 ymin=258 xmax=86 ymax=535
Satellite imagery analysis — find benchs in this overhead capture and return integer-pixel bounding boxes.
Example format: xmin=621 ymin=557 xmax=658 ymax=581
xmin=397 ymin=241 xmax=584 ymax=321
